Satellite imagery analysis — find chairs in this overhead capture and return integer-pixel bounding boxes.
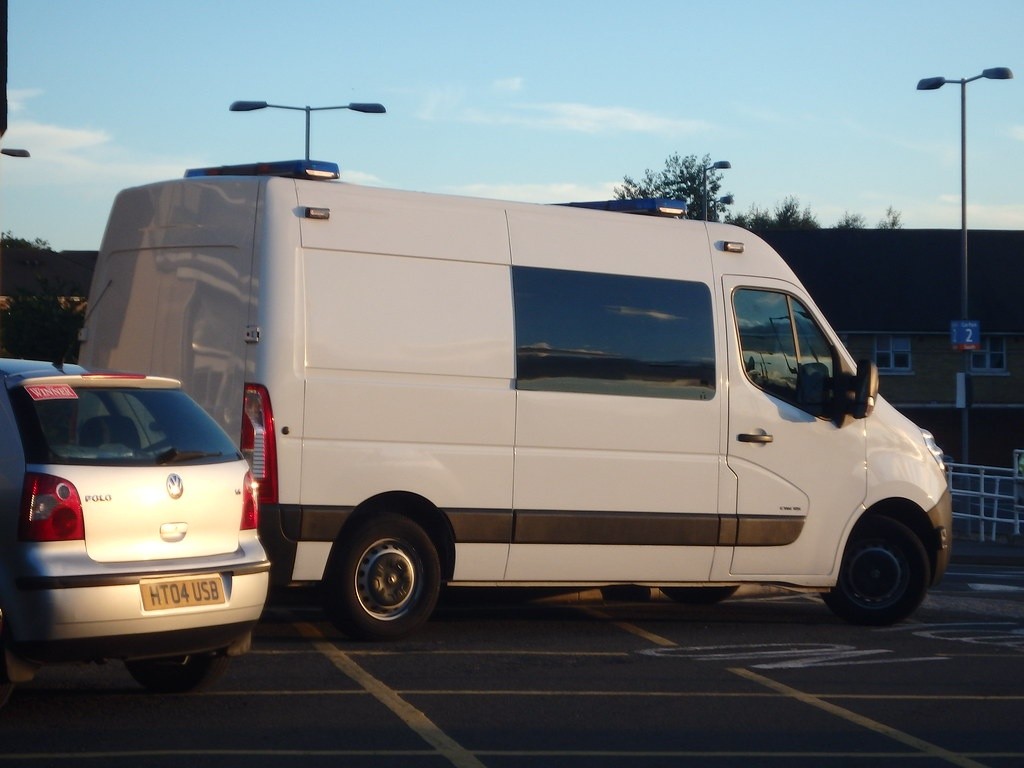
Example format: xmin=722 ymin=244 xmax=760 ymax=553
xmin=80 ymin=416 xmax=144 ymax=453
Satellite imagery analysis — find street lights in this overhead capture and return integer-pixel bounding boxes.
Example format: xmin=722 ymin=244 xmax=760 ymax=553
xmin=704 ymin=161 xmax=731 ymax=221
xmin=229 ymin=99 xmax=387 ymax=158
xmin=713 ymin=196 xmax=732 ymax=224
xmin=916 ymin=67 xmax=1015 ymax=463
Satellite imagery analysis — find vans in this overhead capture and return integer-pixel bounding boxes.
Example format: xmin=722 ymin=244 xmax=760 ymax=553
xmin=81 ymin=177 xmax=953 ymax=636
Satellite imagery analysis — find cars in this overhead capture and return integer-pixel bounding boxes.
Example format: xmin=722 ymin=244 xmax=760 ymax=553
xmin=0 ymin=357 xmax=272 ymax=701
